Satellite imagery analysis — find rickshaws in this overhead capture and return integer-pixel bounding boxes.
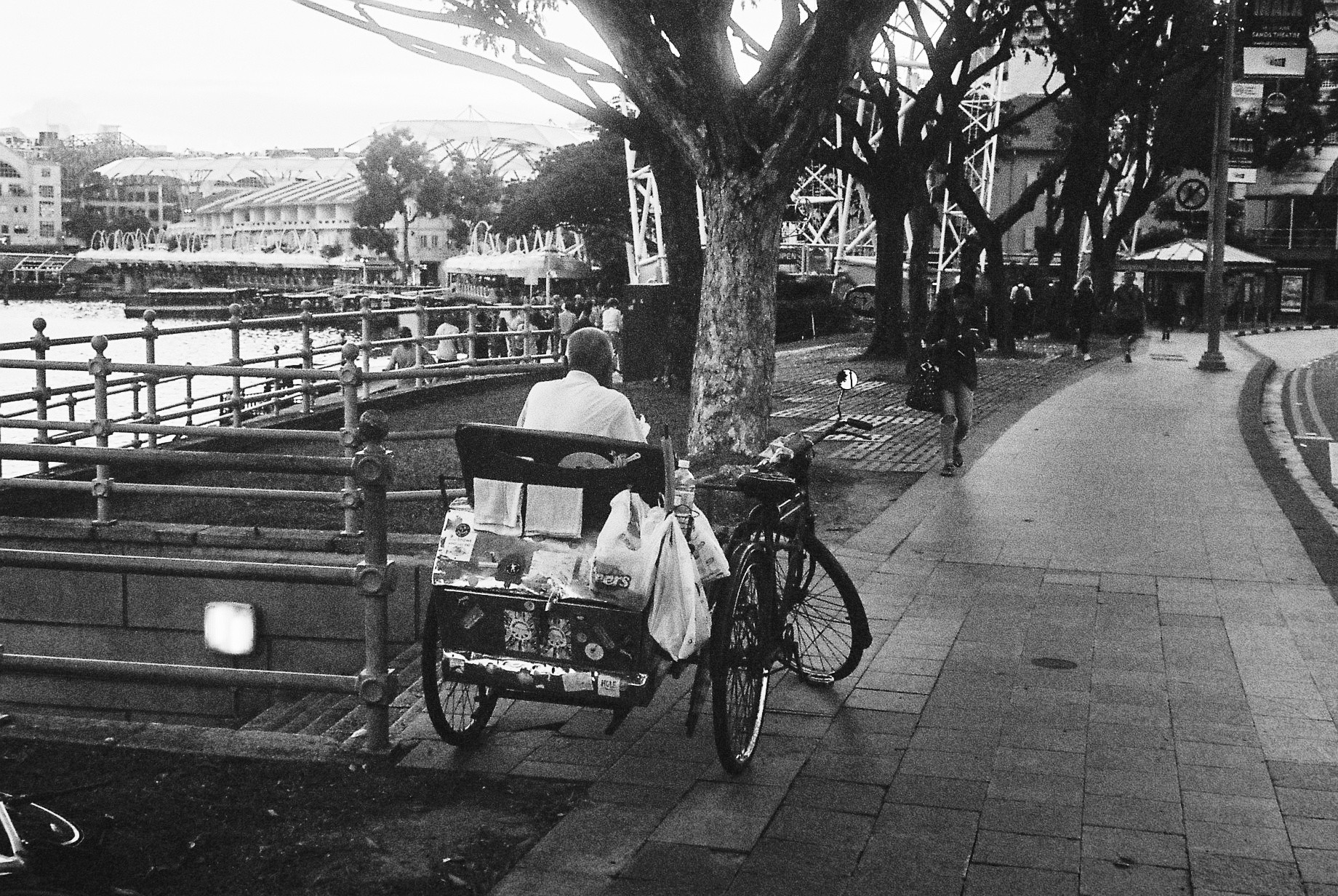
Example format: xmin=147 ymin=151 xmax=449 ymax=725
xmin=418 ymin=369 xmax=875 ymax=772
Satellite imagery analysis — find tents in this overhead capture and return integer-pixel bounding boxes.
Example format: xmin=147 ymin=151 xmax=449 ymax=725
xmin=75 ymin=246 xmax=363 ymax=282
xmin=443 ymin=251 xmax=588 ymax=304
xmin=1119 ymin=236 xmax=1275 ymax=330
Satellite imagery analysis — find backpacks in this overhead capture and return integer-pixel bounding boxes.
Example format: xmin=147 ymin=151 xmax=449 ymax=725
xmin=1015 ymin=285 xmax=1027 ymax=304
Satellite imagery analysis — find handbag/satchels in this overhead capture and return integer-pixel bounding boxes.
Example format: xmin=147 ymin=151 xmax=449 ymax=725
xmin=589 ymin=490 xmax=731 ymax=662
xmin=906 ymin=343 xmax=944 ymax=413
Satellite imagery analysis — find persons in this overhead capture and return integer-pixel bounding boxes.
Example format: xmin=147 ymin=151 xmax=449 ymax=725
xmin=1157 ymin=284 xmax=1179 ymax=341
xmin=1107 ymin=271 xmax=1145 ymax=363
xmin=1071 ymin=275 xmax=1103 ymax=362
xmin=1010 ymin=277 xmax=1033 ymax=341
xmin=383 ymin=290 xmax=624 ymax=389
xmin=919 ymin=281 xmax=991 ymax=477
xmin=515 ymin=326 xmax=648 ymax=445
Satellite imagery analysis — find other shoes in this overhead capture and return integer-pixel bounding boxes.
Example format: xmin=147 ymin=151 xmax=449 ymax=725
xmin=1166 ymin=333 xmax=1170 ymax=340
xmin=1162 ymin=334 xmax=1165 ymax=340
xmin=1084 ymin=353 xmax=1092 ymax=361
xmin=1124 ymin=353 xmax=1132 ymax=363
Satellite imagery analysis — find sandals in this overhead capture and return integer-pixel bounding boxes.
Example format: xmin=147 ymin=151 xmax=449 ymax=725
xmin=940 ymin=463 xmax=954 ymax=477
xmin=952 ymin=446 xmax=965 ymax=468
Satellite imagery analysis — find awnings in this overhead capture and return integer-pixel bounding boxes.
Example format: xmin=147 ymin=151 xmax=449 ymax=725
xmin=1244 ymin=144 xmax=1338 ymax=200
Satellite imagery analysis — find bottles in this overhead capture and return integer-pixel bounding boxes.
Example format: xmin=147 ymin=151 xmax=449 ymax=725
xmin=674 ymin=459 xmax=696 ymax=537
